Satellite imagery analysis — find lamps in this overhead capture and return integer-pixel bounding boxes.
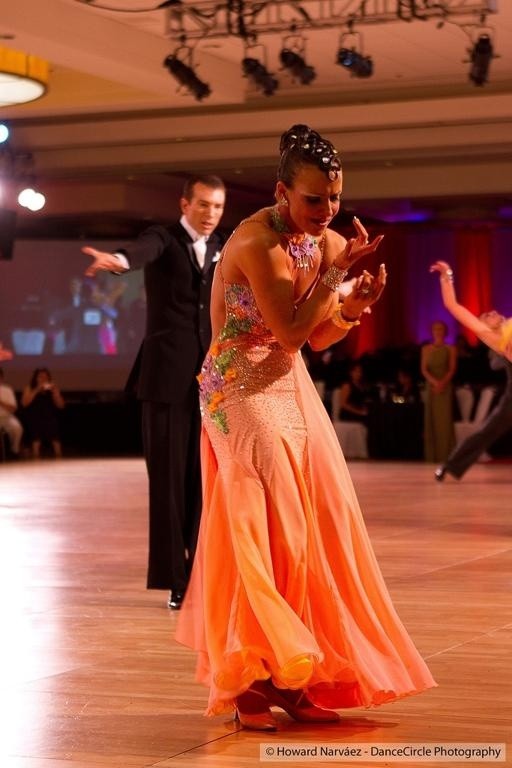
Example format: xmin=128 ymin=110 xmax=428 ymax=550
xmin=0 ymin=176 xmax=53 ymax=216
xmin=0 ymin=0 xmax=512 ymax=109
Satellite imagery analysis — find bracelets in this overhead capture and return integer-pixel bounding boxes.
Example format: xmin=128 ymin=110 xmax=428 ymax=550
xmin=436 ymin=267 xmax=457 ymax=283
xmin=331 ymin=302 xmax=364 ymax=330
xmin=319 ymin=264 xmax=350 ymax=291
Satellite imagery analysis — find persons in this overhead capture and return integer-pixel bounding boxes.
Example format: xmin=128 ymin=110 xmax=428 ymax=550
xmin=453 ymin=329 xmax=502 ymax=424
xmin=170 ymin=119 xmax=444 ymax=731
xmin=335 ymin=362 xmax=383 ymax=460
xmin=426 ymin=254 xmax=512 ymax=366
xmin=383 ymin=372 xmax=425 ymax=460
xmin=0 ymin=363 xmax=24 ymax=459
xmin=419 ymin=320 xmax=459 ymax=464
xmin=80 ymin=168 xmax=236 ymax=608
xmin=19 ymin=367 xmax=67 ymax=456
xmin=429 ymin=379 xmax=510 ymax=482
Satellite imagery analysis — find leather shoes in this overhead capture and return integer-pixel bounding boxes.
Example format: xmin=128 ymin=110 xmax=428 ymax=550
xmin=434 ymin=466 xmax=445 ymax=480
xmin=169 ymin=589 xmax=183 ymax=609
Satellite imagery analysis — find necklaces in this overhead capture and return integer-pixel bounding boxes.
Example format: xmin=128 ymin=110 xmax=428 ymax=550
xmin=275 ymin=209 xmax=325 ymax=272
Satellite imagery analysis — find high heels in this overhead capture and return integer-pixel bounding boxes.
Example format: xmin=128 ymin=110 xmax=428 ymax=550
xmin=231 ymin=688 xmax=279 ymax=730
xmin=267 ymin=686 xmax=340 ymax=723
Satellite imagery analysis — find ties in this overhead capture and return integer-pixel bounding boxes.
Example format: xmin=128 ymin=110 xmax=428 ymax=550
xmin=193 ymin=235 xmax=207 ymax=269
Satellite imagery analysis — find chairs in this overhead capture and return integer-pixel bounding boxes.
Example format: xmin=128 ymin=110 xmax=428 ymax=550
xmin=303 ymin=336 xmax=512 ymax=475
